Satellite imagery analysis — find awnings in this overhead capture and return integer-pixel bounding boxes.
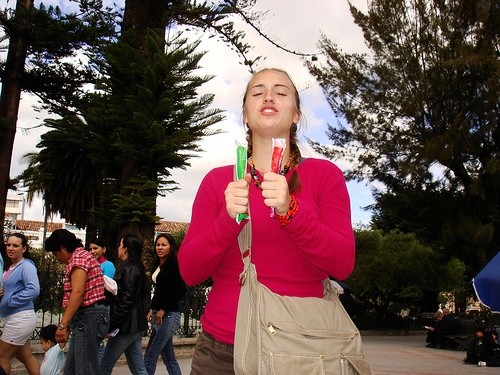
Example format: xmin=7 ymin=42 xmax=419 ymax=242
xmin=470 ymin=250 xmax=500 ymax=315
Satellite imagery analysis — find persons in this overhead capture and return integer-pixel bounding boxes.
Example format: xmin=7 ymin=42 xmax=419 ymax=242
xmin=143 ymin=234 xmax=187 ymax=375
xmin=39 ymin=324 xmax=65 ymax=375
xmin=178 ymin=69 xmax=355 ymax=375
xmin=0 ymin=233 xmax=40 ymax=375
xmin=425 ymin=309 xmax=500 ymax=366
xmin=45 ymin=229 xmax=110 ymax=375
xmin=88 ymin=239 xmax=116 ymax=279
xmin=100 ymin=234 xmax=148 ymax=375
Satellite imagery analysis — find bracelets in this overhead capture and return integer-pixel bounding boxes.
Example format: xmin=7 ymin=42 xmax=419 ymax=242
xmin=273 ymin=199 xmax=298 ymax=228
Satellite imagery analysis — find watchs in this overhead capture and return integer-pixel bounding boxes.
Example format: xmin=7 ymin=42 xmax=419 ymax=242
xmin=59 ymin=324 xmax=65 ymax=330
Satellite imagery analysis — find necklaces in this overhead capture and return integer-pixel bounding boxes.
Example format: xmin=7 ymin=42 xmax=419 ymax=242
xmin=250 ymin=154 xmax=294 ymax=189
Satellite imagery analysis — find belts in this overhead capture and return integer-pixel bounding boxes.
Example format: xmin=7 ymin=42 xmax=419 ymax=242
xmin=79 ymin=301 xmax=103 ymax=310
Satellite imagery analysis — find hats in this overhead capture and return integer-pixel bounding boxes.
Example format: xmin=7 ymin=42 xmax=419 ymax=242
xmin=434 ymin=312 xmax=442 ymax=318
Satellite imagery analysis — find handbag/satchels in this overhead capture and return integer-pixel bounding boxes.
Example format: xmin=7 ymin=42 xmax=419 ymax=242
xmin=233 ymin=261 xmax=373 ymax=375
